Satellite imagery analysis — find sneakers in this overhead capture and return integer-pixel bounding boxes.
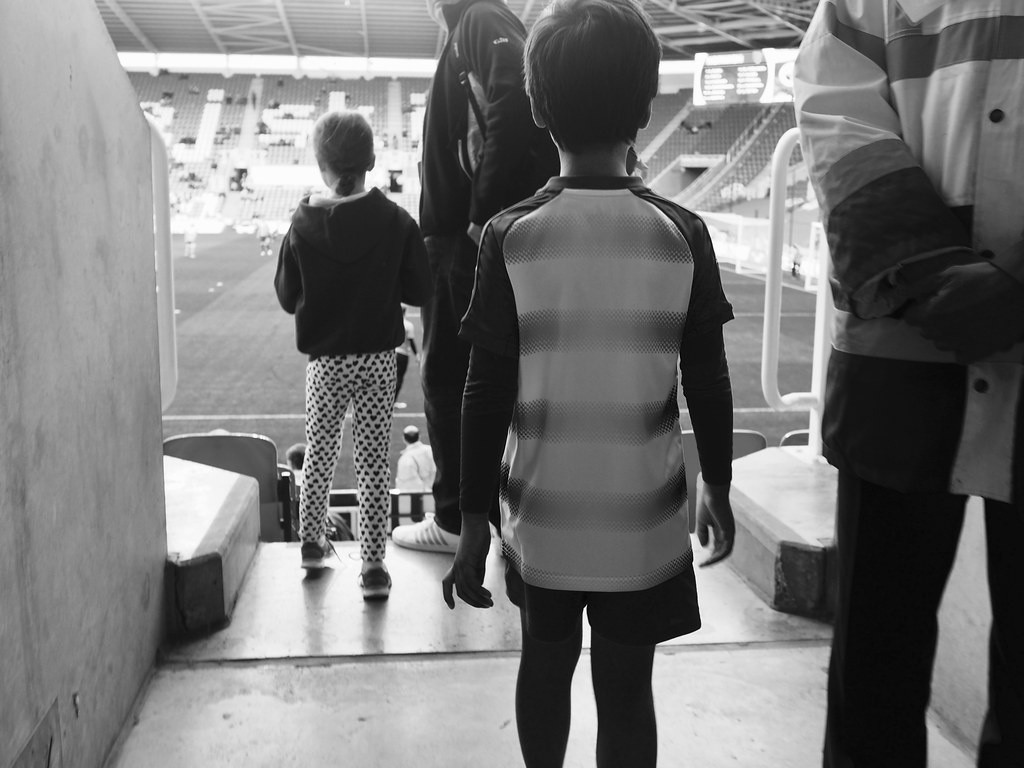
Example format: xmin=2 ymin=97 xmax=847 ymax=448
xmin=392 ymin=512 xmax=460 ymax=553
xmin=301 ymin=537 xmax=339 ymax=568
xmin=359 ymin=567 xmax=392 ymax=599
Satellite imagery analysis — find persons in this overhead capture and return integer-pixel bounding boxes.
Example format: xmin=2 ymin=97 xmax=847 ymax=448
xmin=789 ymin=1 xmax=1024 ymax=768
xmin=390 ymin=0 xmax=561 ymax=557
xmin=274 ymin=109 xmax=433 ymax=600
xmin=443 ymin=0 xmax=735 ymax=768
xmin=393 ymin=425 xmax=436 ymax=492
xmin=283 ymin=442 xmax=309 ymax=487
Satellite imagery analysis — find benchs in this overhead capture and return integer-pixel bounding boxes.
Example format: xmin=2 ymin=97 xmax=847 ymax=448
xmin=158 ymin=426 xmax=812 ymax=544
